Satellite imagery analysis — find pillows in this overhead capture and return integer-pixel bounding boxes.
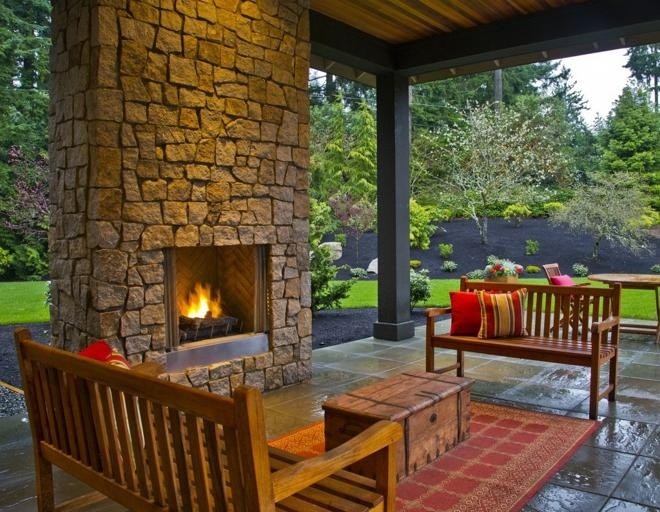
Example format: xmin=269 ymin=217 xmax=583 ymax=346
xmin=476 ymin=288 xmax=529 ymax=339
xmin=553 ymin=274 xmax=575 ymax=285
xmin=79 ymin=342 xmax=156 ymax=449
xmin=448 ymin=290 xmax=501 ymax=336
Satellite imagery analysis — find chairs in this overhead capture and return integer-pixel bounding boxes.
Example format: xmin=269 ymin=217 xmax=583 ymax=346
xmin=424 ymin=276 xmax=622 ymax=416
xmin=542 ymin=262 xmax=598 ymax=335
xmin=16 ymin=329 xmax=404 ymax=511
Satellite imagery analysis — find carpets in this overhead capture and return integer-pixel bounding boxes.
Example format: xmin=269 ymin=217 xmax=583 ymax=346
xmin=267 ymin=399 xmax=602 ymax=512
